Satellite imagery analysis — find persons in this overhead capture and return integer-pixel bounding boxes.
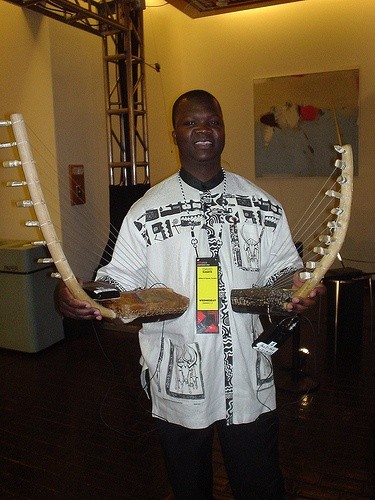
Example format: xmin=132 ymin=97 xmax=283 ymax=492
xmin=56 ymin=90 xmax=326 ymax=500
